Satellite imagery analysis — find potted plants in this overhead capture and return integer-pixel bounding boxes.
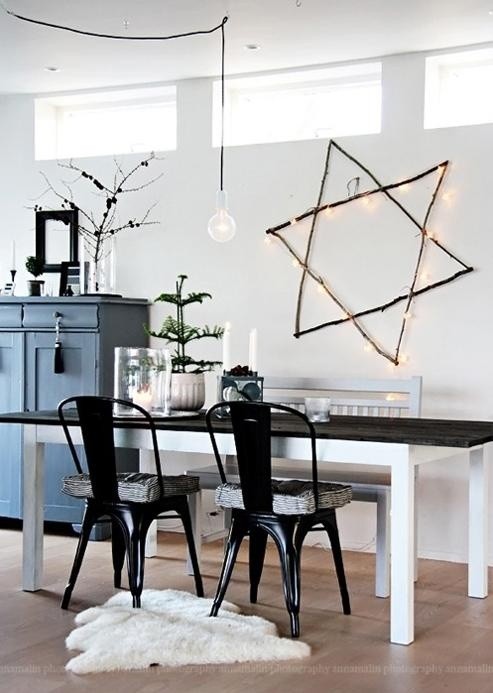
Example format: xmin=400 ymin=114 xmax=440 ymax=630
xmin=141 ymin=274 xmax=223 ymax=409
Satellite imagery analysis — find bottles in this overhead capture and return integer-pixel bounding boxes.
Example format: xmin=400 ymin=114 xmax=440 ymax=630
xmin=66 ymin=285 xmax=73 ymax=297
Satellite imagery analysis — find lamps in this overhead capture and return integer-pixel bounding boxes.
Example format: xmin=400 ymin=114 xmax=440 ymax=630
xmin=0 ymin=1 xmax=239 ymax=241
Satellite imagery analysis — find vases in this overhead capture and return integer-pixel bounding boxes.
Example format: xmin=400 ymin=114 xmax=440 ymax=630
xmin=77 ymin=234 xmax=115 ymax=295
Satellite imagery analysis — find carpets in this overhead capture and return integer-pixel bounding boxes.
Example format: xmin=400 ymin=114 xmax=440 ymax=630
xmin=65 ymin=587 xmax=311 ymax=675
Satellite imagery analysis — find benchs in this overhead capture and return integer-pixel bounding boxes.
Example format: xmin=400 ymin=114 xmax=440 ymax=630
xmin=185 ymin=377 xmax=422 ymax=598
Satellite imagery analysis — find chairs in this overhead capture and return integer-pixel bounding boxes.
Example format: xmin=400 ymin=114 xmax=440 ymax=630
xmin=55 ymin=395 xmax=204 ymax=610
xmin=205 ymin=400 xmax=355 ymax=639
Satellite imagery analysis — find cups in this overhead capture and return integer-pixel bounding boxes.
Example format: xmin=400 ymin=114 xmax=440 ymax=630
xmin=81 ymin=232 xmax=116 ymax=294
xmin=304 ymin=398 xmax=331 ymax=424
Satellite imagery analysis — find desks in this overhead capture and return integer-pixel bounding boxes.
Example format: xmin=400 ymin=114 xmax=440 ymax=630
xmin=0 ymin=404 xmax=493 ymax=646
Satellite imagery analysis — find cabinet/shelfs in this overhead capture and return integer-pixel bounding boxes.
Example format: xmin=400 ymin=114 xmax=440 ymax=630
xmin=0 ymin=295 xmax=152 ymax=541
